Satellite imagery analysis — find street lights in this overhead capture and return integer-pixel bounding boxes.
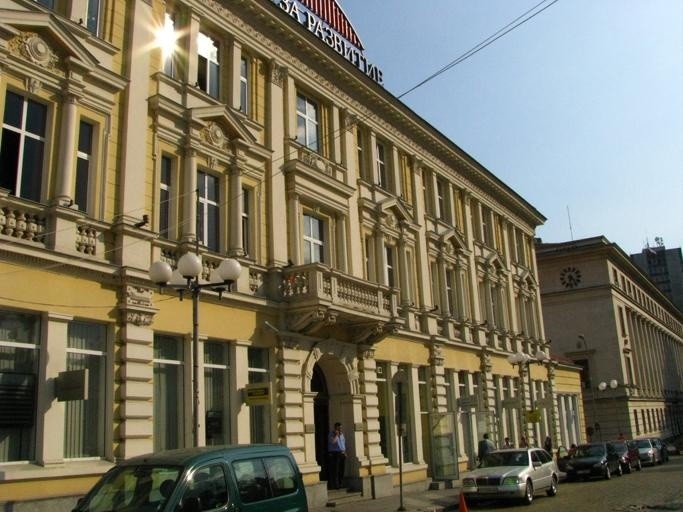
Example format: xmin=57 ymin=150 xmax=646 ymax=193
xmin=149 ymin=252 xmax=242 ymax=450
xmin=598 ymin=379 xmax=624 ymax=438
xmin=508 ymin=351 xmax=545 ymax=447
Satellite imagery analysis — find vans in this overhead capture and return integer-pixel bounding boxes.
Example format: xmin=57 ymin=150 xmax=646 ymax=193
xmin=70 ymin=443 xmax=309 ymax=512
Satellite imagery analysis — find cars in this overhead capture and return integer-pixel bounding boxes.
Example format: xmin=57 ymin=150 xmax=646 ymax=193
xmin=461 ymin=437 xmax=680 ymax=505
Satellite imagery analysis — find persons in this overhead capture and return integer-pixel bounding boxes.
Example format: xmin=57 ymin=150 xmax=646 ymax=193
xmin=327 ymin=421 xmax=346 ymax=489
xmin=519 ymin=437 xmax=530 ymax=448
xmin=567 ymin=443 xmax=577 ymax=455
xmin=501 ymin=436 xmax=513 ymax=448
xmin=476 ymin=432 xmax=495 ymax=459
xmin=616 ymin=432 xmax=624 ymax=440
xmin=544 ymin=436 xmax=551 ymax=452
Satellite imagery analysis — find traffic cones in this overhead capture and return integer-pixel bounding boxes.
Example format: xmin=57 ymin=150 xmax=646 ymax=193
xmin=458 ymin=491 xmax=468 ymax=512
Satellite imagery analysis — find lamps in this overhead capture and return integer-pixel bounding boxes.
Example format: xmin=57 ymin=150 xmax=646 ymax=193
xmin=135 ymin=215 xmax=149 ymax=228
xmin=430 ymin=305 xmax=552 ymax=344
xmin=284 ymin=259 xmax=294 ymax=268
xmin=68 ymin=199 xmax=73 ymax=206
